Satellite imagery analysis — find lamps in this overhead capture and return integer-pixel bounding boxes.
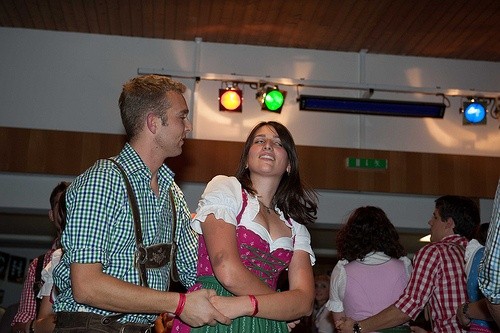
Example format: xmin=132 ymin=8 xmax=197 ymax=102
xmin=256 ymin=86 xmax=287 ymax=114
xmin=459 ymin=99 xmax=488 ymax=126
xmin=219 ymin=83 xmax=243 ymax=112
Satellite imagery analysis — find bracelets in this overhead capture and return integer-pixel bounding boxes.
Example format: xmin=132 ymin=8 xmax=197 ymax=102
xmin=462 ymin=302 xmax=472 ymax=319
xmin=248 ymin=294 xmax=259 ymax=316
xmin=174 ymin=292 xmax=187 ymax=316
xmin=29 ymin=319 xmax=35 ymax=332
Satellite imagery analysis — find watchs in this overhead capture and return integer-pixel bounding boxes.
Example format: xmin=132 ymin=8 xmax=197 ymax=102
xmin=353 ymin=321 xmax=362 ymax=333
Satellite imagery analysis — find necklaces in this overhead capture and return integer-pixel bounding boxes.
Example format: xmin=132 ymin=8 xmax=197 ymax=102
xmin=259 ymin=200 xmax=272 ymax=213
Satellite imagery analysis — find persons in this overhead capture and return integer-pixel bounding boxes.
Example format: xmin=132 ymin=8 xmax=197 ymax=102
xmin=50 ymin=73 xmax=300 ymax=332
xmin=456 ymin=231 xmax=496 ymax=333
xmin=36 ymin=190 xmax=67 ymax=319
xmin=476 ymin=175 xmax=500 ymax=333
xmin=169 ymin=120 xmax=316 ymax=333
xmin=10 ymin=181 xmax=72 ymax=333
xmin=335 ymin=194 xmax=481 ymax=333
xmin=311 ymin=273 xmax=336 ymax=332
xmin=325 ymin=205 xmax=413 ymax=333
